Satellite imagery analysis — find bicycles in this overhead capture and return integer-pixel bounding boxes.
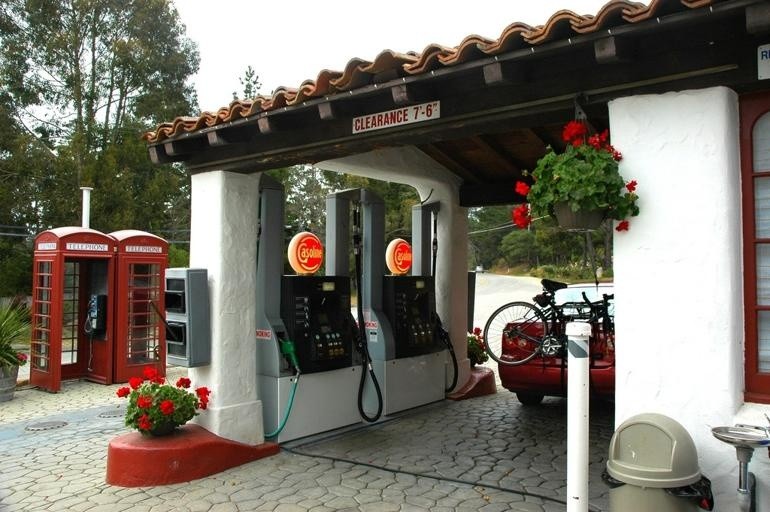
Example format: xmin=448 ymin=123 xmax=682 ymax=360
xmin=483 ymin=280 xmax=615 ymax=368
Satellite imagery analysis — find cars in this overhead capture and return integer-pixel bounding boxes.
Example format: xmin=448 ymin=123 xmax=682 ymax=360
xmin=498 ymin=282 xmax=615 ymax=405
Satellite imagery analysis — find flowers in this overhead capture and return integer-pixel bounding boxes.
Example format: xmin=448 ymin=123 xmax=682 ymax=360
xmin=0 ymin=294 xmax=36 ymax=375
xmin=511 ymin=119 xmax=640 ymax=231
xmin=116 ymin=365 xmax=211 ymax=437
xmin=467 ymin=327 xmax=489 ymax=364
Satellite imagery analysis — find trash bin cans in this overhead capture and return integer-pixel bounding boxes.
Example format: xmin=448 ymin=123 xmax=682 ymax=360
xmin=607 ymin=414 xmax=701 ymax=512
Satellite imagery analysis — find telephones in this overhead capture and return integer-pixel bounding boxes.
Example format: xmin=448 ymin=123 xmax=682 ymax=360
xmin=88 ymin=294 xmax=106 ymax=334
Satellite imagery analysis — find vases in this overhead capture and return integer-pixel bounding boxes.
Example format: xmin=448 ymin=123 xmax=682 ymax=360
xmin=468 ymin=354 xmax=477 ymax=369
xmin=0 ymin=364 xmax=18 ymax=403
xmin=149 ymin=423 xmax=174 ymax=437
xmin=553 ymin=202 xmax=605 ymax=232
